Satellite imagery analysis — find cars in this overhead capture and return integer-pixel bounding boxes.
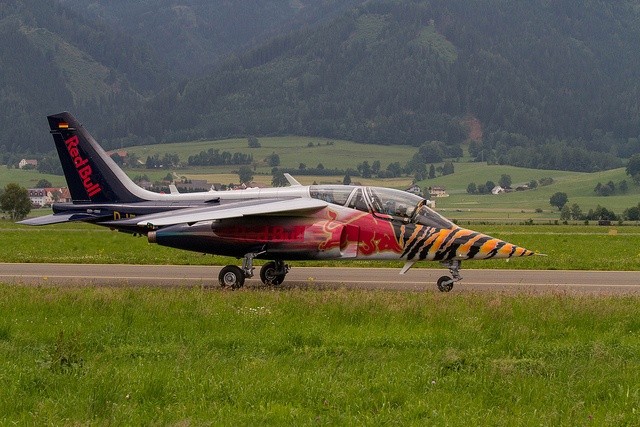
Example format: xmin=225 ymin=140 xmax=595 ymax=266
xmin=492 ymin=185 xmax=513 ymax=195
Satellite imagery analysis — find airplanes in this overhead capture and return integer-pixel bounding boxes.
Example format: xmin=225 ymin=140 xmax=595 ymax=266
xmin=15 ymin=112 xmax=546 ymax=292
